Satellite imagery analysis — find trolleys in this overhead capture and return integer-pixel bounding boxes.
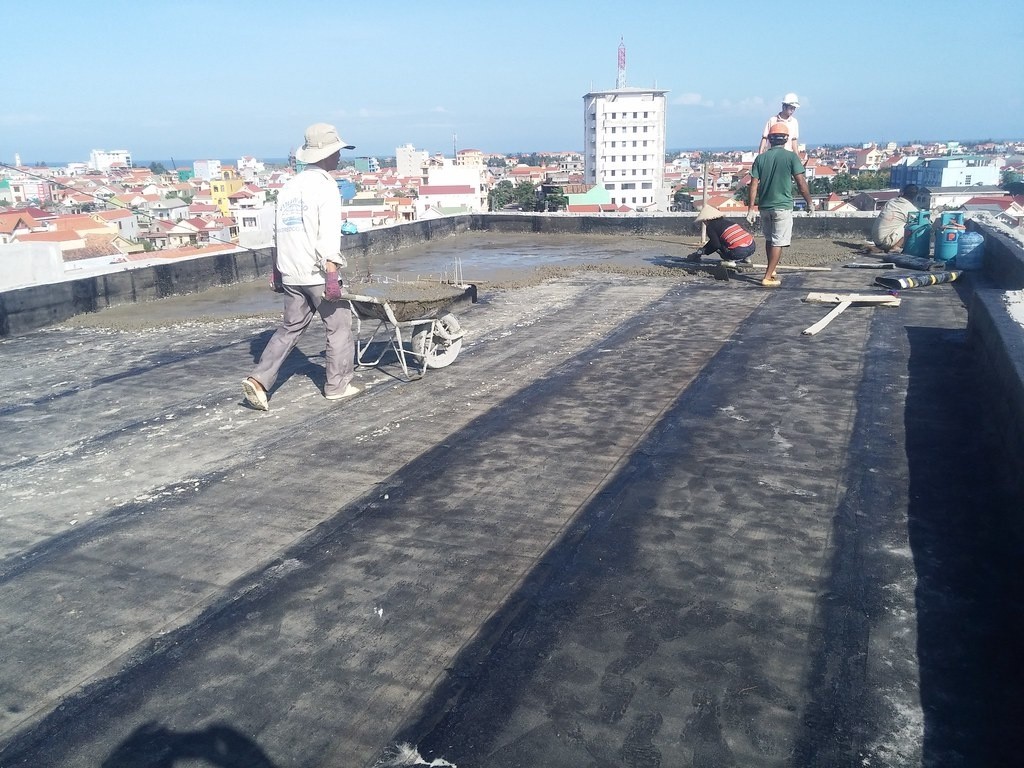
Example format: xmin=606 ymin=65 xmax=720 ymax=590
xmin=267 ymin=274 xmax=478 ymax=382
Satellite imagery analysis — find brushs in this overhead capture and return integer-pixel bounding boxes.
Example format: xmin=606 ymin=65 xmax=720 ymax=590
xmin=688 ymin=252 xmax=704 ymax=263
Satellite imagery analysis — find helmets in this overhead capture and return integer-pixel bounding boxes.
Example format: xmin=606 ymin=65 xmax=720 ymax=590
xmin=781 ymin=93 xmax=800 ymax=108
xmin=768 ymin=122 xmax=789 ymax=137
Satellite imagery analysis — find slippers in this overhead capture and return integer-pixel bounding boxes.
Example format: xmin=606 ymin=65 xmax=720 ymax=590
xmin=240 ymin=379 xmax=269 ymax=411
xmin=325 ymin=383 xmax=360 ymax=400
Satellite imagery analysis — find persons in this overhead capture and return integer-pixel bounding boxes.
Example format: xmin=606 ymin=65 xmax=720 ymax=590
xmin=747 ymin=122 xmax=815 ymax=286
xmin=686 ymin=204 xmax=755 ymax=267
xmin=759 ymin=93 xmax=801 ymax=158
xmin=872 ymin=184 xmax=921 ymax=254
xmin=239 ymin=123 xmax=360 ymax=410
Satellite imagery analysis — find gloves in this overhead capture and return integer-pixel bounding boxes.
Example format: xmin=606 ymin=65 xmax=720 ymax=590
xmin=745 ymin=209 xmax=757 ymax=227
xmin=268 ymin=264 xmax=284 ymax=293
xmin=805 ymin=204 xmax=816 ymax=216
xmin=323 ymin=271 xmax=342 ymax=304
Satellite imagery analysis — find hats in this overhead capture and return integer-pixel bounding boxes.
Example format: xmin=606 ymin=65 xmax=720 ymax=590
xmin=294 ymin=122 xmax=356 ymax=164
xmin=692 ymin=203 xmax=725 ymax=223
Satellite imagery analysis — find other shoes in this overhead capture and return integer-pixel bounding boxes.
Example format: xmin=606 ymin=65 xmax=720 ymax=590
xmin=720 ymin=260 xmax=736 ymax=268
xmin=888 ymin=246 xmax=904 ymax=254
xmin=761 ymin=278 xmax=781 ymax=287
xmin=744 ymin=257 xmax=752 ymax=263
xmin=771 ymin=271 xmax=777 ymax=276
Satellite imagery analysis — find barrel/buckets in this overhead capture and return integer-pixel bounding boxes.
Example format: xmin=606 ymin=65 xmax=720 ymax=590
xmin=956 ymin=231 xmax=986 ymax=269
